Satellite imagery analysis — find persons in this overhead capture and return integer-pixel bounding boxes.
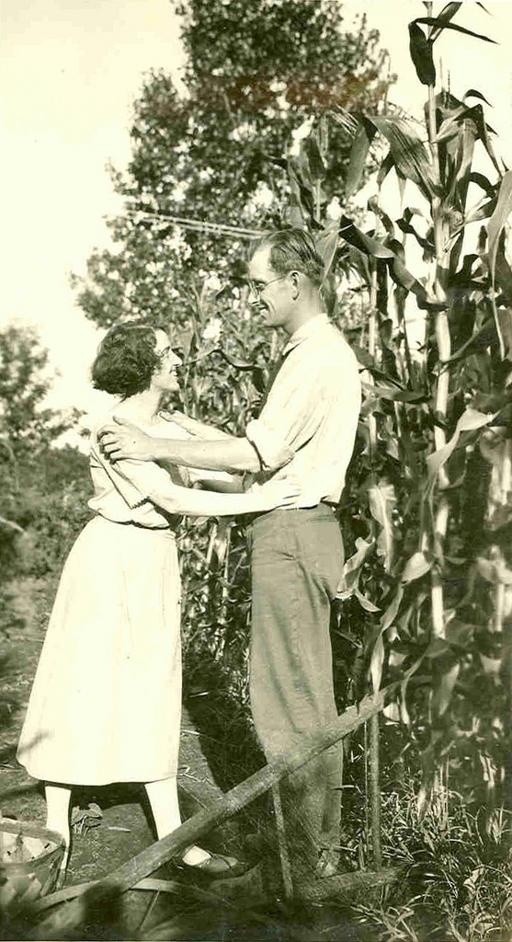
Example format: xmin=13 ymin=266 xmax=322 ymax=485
xmin=93 ymin=224 xmax=363 ymax=905
xmin=17 ymin=314 xmax=309 ymax=901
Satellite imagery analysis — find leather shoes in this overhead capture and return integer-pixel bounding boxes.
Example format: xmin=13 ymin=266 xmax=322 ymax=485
xmin=164 ymin=841 xmax=253 ymax=879
xmin=56 ymin=845 xmax=70 ymax=889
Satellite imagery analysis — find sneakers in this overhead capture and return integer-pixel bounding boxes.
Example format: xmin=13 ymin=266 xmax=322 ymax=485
xmin=243 ymin=833 xmax=339 ymax=878
xmin=209 ymin=859 xmax=342 ymax=903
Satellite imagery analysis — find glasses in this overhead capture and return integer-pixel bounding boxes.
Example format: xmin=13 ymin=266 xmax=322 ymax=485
xmin=248 ymin=276 xmax=286 ymax=299
